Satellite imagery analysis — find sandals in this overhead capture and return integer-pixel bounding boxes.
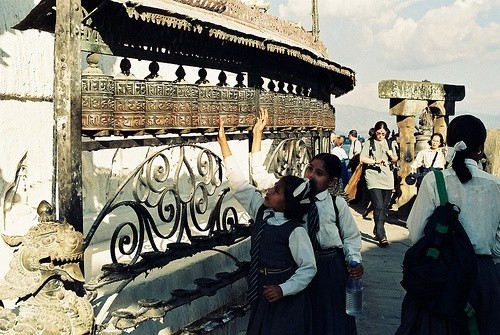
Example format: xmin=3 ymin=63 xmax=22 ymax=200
xmin=373 ymin=228 xmax=379 ymax=239
xmin=379 ymin=239 xmax=388 ymax=246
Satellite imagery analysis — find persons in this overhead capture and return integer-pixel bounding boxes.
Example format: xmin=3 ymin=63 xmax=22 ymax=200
xmin=397 ymin=115 xmax=500 ymax=335
xmin=360 ymin=121 xmax=399 ymax=249
xmin=216 ymin=117 xmax=318 ymax=335
xmin=326 ymin=129 xmax=448 ymax=187
xmin=249 ymin=107 xmax=364 ymax=335
xmin=477 ymin=153 xmax=488 ymax=172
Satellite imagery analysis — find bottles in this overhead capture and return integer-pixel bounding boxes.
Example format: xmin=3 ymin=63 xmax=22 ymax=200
xmin=345 ymin=261 xmax=364 ymax=316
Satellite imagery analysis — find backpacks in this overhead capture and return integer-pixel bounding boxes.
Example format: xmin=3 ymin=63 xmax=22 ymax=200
xmin=399 ymin=202 xmax=477 ymax=321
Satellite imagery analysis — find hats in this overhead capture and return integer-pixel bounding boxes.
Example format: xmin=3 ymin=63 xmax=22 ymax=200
xmin=405 ymin=173 xmax=416 ymax=185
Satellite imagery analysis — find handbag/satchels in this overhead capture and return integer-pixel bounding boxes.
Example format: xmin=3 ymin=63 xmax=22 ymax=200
xmin=415 ymin=175 xmax=424 ymax=187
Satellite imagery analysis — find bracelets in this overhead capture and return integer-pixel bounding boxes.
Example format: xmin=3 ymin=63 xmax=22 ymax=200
xmin=374 ymin=159 xmax=377 ymax=165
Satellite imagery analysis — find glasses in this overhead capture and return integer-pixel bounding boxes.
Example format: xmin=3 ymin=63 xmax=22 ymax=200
xmin=376 ymin=132 xmax=386 ymax=136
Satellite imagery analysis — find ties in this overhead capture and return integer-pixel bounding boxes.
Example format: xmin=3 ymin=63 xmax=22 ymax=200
xmin=307 ymin=196 xmax=320 ymax=264
xmin=247 ymin=211 xmax=275 ymax=305
